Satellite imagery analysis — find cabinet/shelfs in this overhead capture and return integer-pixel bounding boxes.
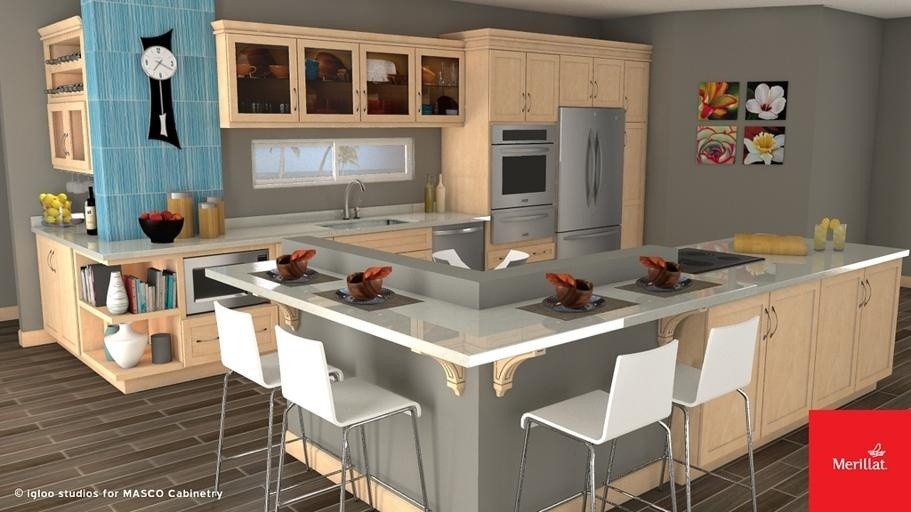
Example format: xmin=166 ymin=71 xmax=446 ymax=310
xmin=297 ymin=40 xmax=415 ymax=123
xmin=697 ymin=277 xmax=821 ymax=467
xmin=415 ymin=48 xmax=465 ymax=122
xmin=38 ymin=16 xmax=92 ymax=174
xmin=560 ymin=55 xmax=625 ymax=108
xmin=487 ymin=241 xmax=555 ymax=270
xmin=335 ymin=228 xmax=433 ymax=261
xmin=620 ymin=61 xmax=650 ymax=251
xmin=32 ymin=232 xmax=277 ymax=396
xmin=227 ymin=33 xmax=299 ymax=122
xmin=811 ymin=260 xmax=903 ymax=410
xmin=489 ymin=49 xmax=560 ymax=124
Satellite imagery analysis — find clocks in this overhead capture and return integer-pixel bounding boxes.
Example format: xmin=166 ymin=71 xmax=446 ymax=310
xmin=141 ymin=46 xmax=177 ymax=80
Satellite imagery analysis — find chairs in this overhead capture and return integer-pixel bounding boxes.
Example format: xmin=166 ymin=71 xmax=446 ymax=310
xmin=659 ymin=314 xmax=759 ymax=512
xmin=272 ymin=324 xmax=429 ymax=512
xmin=514 ymin=339 xmax=679 ymax=512
xmin=213 ymin=301 xmax=358 ymax=512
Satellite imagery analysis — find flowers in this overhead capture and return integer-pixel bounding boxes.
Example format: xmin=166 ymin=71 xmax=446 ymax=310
xmin=695 ymin=80 xmax=785 ymax=165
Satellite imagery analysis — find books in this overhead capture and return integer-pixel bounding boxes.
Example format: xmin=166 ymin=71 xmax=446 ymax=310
xmin=80 ymin=263 xmax=177 ymax=314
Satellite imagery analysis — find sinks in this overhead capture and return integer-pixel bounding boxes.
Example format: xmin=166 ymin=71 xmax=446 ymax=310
xmin=317 ymin=216 xmax=408 ymax=232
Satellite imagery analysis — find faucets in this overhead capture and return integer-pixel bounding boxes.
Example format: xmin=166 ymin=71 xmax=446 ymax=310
xmin=341 ymin=178 xmax=367 ymax=220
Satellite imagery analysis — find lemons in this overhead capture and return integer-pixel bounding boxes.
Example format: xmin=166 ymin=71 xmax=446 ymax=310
xmin=38 ymin=192 xmax=72 ymax=225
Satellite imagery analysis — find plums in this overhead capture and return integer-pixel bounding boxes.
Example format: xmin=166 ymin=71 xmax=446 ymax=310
xmin=139 ymin=209 xmax=183 ymax=222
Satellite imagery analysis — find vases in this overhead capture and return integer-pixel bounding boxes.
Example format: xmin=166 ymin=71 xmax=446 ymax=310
xmin=104 ymin=322 xmax=149 ymax=369
xmin=106 ymin=272 xmax=129 ymax=315
